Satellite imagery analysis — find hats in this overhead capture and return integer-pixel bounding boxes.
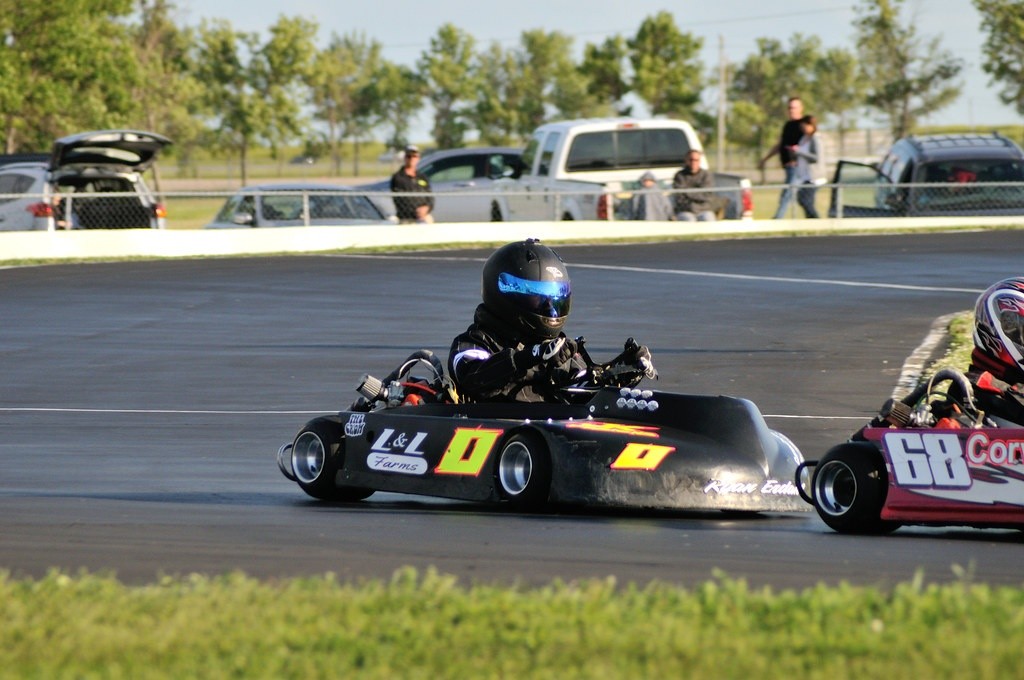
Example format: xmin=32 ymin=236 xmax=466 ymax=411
xmin=406 ymin=144 xmax=420 ymax=154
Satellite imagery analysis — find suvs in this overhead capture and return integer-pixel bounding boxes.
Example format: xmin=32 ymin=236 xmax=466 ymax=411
xmin=0 ymin=129 xmax=174 ymax=231
xmin=827 ymin=131 xmax=1024 ymax=221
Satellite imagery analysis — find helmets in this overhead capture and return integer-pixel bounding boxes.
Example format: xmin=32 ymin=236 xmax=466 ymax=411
xmin=480 ymin=238 xmax=573 ymax=341
xmin=970 ymin=276 xmax=1024 ymax=379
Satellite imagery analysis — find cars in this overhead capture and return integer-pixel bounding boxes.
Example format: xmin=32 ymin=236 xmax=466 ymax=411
xmin=346 ymin=146 xmax=549 ymax=224
xmin=203 ymin=181 xmax=400 ymax=227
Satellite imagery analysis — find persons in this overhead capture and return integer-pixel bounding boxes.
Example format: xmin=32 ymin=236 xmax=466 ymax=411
xmin=948 ymin=276 xmax=1024 ymax=425
xmin=671 ymin=151 xmax=716 ymax=221
xmin=390 ymin=146 xmax=435 ymax=224
xmin=756 ymin=97 xmax=823 ymax=220
xmin=631 ymin=172 xmax=672 ymax=221
xmin=448 ymin=238 xmax=652 ymax=406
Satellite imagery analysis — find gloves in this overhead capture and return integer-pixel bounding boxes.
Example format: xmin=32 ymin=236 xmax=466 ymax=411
xmin=637 ymin=343 xmax=655 ymax=376
xmin=524 ymin=337 xmax=580 ymax=367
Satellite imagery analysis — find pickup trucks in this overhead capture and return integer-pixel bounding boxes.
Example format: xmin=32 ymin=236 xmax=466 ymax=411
xmin=521 ymin=117 xmax=753 ymax=222
